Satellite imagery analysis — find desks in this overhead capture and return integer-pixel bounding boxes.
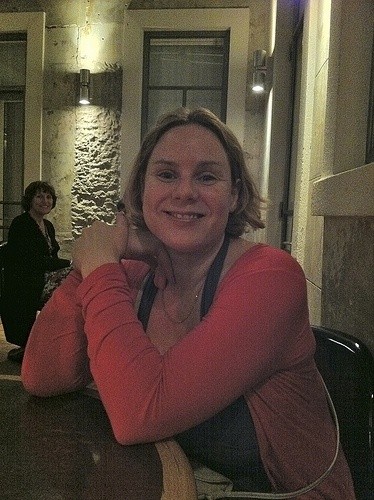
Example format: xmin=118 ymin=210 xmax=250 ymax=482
xmin=0 ymin=375 xmax=197 ymax=500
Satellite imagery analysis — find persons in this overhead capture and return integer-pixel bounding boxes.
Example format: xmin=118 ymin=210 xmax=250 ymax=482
xmin=22 ymin=105 xmax=356 ymax=500
xmin=0 ymin=182 xmax=73 ymax=350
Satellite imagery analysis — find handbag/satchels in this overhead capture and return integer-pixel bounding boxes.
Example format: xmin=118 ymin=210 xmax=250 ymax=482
xmin=186 ymin=456 xmax=234 ymax=500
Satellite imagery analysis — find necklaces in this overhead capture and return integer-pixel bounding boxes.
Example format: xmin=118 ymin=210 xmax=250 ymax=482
xmin=162 ymin=281 xmax=205 ymax=325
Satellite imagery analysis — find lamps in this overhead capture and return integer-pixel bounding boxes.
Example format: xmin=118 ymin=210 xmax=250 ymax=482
xmin=79 ymin=69 xmax=91 ymax=104
xmin=251 ymin=50 xmax=267 ymax=91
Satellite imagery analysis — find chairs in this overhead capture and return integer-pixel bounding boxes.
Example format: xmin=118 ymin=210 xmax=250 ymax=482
xmin=0 ymin=241 xmax=33 ymax=363
xmin=311 ymin=325 xmax=374 ymax=500
